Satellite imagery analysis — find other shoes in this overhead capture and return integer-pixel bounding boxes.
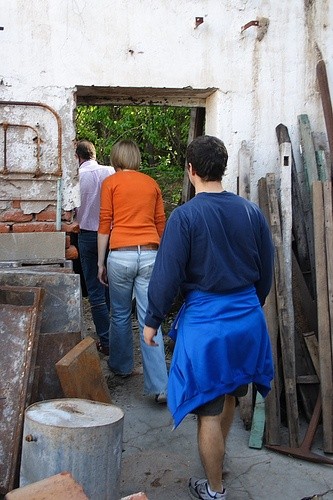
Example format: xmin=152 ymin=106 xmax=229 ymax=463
xmin=144 ymin=393 xmax=167 ymax=403
xmin=96 ymin=341 xmax=109 ymax=356
xmin=107 ymin=359 xmax=127 ymax=377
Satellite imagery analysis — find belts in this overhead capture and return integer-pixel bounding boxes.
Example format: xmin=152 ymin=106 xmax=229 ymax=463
xmin=111 ymin=242 xmax=159 ymax=251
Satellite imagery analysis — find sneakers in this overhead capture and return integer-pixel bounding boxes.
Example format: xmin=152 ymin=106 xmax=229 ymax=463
xmin=222 ymin=455 xmax=231 ymax=474
xmin=187 ymin=477 xmax=227 ymax=500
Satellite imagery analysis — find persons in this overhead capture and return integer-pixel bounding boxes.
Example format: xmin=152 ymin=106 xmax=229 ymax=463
xmin=143 ymin=135 xmax=274 ymax=500
xmin=96 ymin=139 xmax=172 ymax=406
xmin=65 ymin=139 xmax=116 ymax=356
